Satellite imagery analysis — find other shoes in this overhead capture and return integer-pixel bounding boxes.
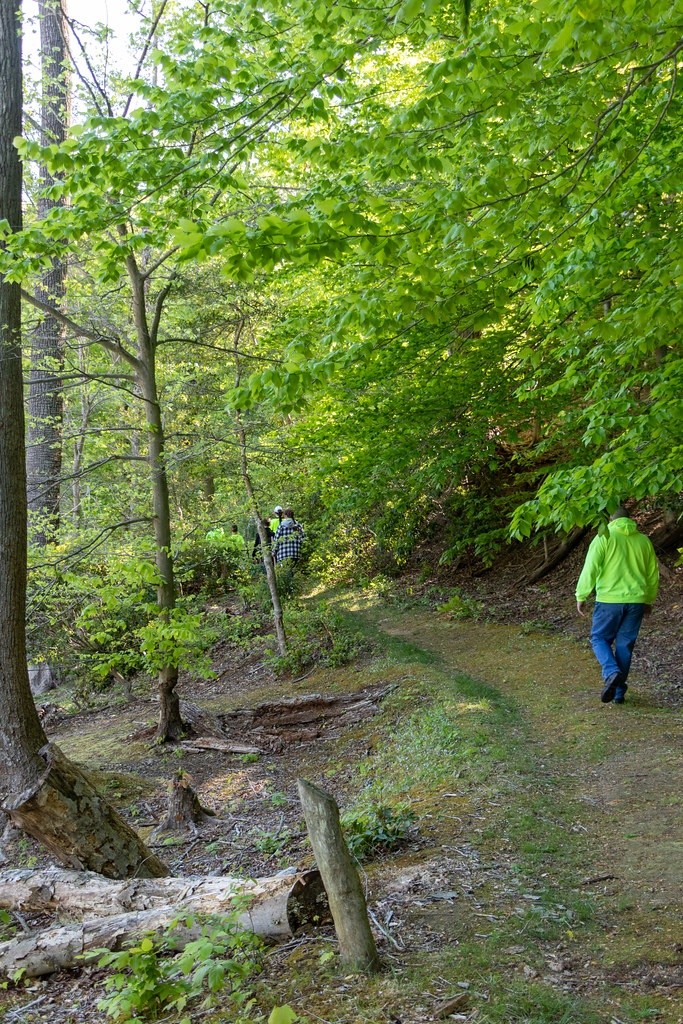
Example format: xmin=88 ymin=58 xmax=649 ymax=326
xmin=600 ymin=672 xmax=622 ymax=703
xmin=615 ymin=698 xmax=625 ymax=704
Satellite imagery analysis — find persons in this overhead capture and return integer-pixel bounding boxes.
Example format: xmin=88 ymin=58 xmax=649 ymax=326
xmin=271 ymin=508 xmax=307 ymax=597
xmin=574 ymin=502 xmax=660 ymax=704
xmin=269 ymin=505 xmax=286 ymax=534
xmin=251 ymin=519 xmax=276 ymax=564
xmin=230 ymin=525 xmax=237 ymax=535
